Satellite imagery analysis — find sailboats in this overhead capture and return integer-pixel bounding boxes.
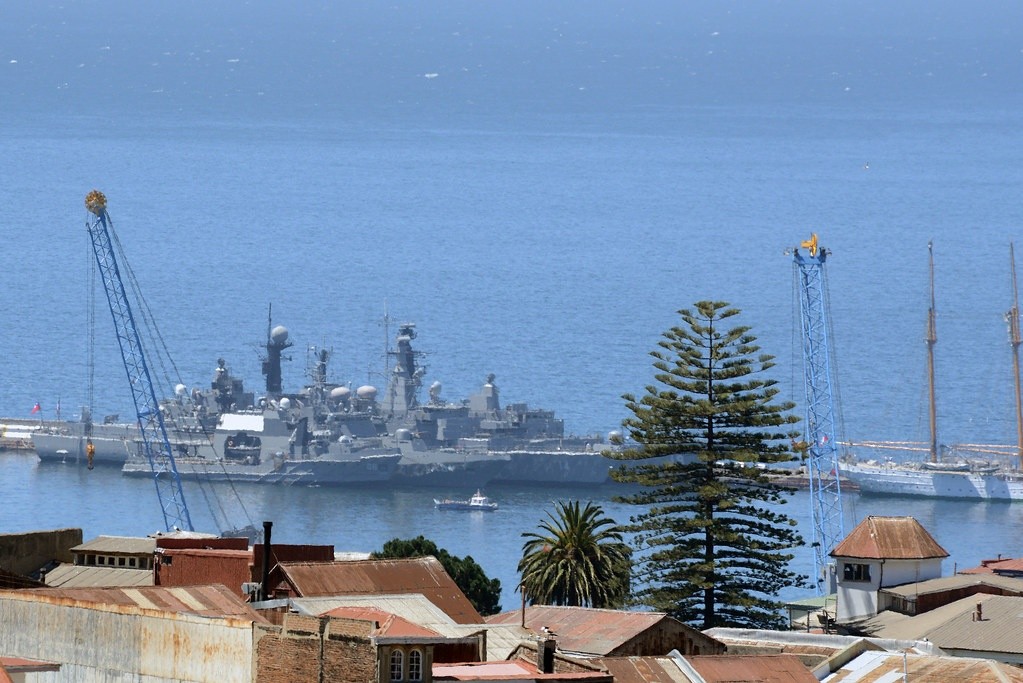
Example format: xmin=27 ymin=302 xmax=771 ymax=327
xmin=831 ymin=241 xmax=1023 ymax=502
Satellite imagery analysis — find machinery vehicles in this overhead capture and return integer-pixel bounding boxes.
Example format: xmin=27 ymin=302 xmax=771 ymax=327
xmin=84 ymin=191 xmax=259 ymax=546
xmin=792 ymin=234 xmax=857 ymax=596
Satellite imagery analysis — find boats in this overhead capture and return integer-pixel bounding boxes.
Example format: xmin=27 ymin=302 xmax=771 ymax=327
xmin=461 ymin=374 xmax=612 ymax=484
xmin=284 ymin=323 xmax=513 ymax=490
xmin=120 ymin=301 xmax=402 ymax=481
xmin=433 ymin=489 xmax=498 ymax=512
xmin=30 ymin=423 xmax=162 ymax=461
